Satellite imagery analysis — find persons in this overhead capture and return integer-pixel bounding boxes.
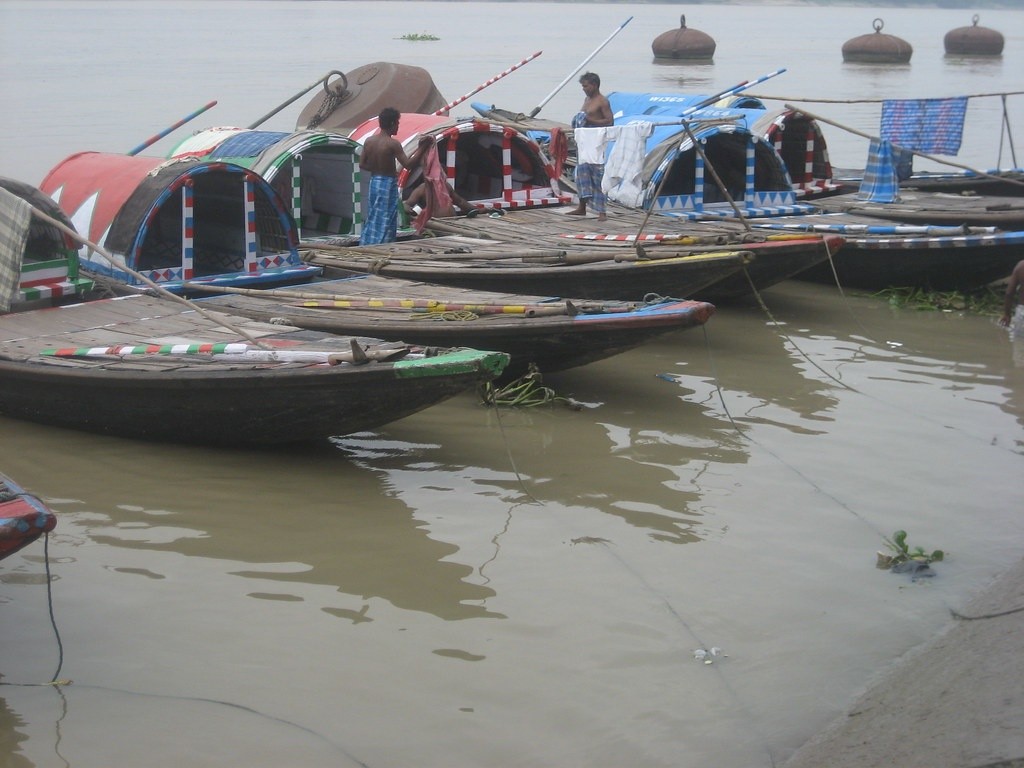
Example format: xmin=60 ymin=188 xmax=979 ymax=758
xmin=999 ymin=260 xmax=1024 ymax=336
xmin=564 ymin=72 xmax=614 ymax=222
xmin=359 ymin=108 xmax=433 ymax=246
xmin=403 ymin=163 xmax=503 ymax=219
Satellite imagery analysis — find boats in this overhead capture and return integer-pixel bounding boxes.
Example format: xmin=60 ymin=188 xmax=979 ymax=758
xmin=32 ymin=150 xmax=717 ymax=378
xmin=0 ymin=175 xmax=511 ymax=448
xmin=468 ymin=89 xmax=1024 ymax=289
xmin=171 ymin=125 xmax=755 ymax=303
xmin=342 ymin=111 xmax=846 ymax=301
